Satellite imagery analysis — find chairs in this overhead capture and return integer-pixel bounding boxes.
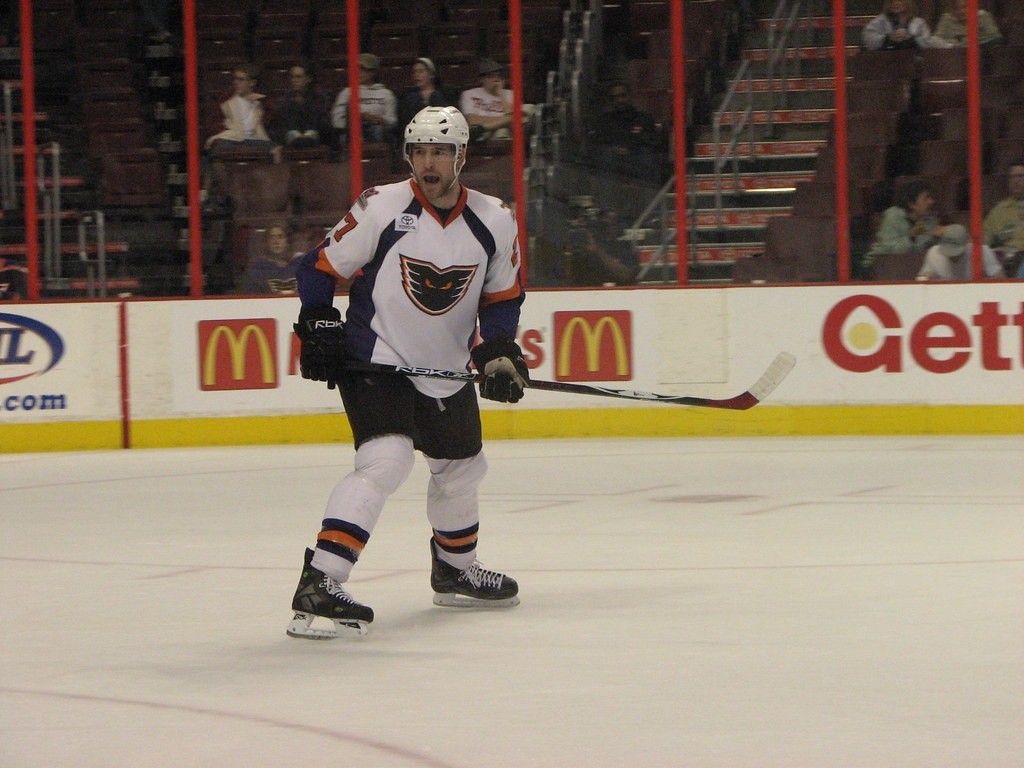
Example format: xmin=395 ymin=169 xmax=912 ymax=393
xmin=733 ymin=0 xmax=1024 ymax=281
xmin=25 ymin=0 xmax=735 ymax=298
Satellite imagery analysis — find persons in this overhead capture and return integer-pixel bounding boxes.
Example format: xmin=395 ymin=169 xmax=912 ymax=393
xmin=332 ymin=52 xmax=398 ymax=143
xmin=869 ymin=162 xmax=1024 ymax=282
xmin=397 ymin=56 xmax=458 ymax=143
xmin=861 ymin=0 xmax=1005 ymax=51
xmin=295 ymin=107 xmax=530 ymax=639
xmin=459 ymin=58 xmax=529 ymax=142
xmin=547 ymin=195 xmax=639 ymax=285
xmin=237 ymin=223 xmax=298 ymax=294
xmin=585 ymin=81 xmax=658 ymax=227
xmin=200 ymin=62 xmax=283 ymax=200
xmin=268 ymin=58 xmax=339 ymax=151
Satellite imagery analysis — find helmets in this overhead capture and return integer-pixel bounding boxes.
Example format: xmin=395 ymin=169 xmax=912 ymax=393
xmin=403 ymin=105 xmax=469 ymax=165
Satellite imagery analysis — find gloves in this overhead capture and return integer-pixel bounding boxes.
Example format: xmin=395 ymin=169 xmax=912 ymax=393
xmin=292 ymin=307 xmax=347 ymax=390
xmin=470 ymin=338 xmax=530 ymax=403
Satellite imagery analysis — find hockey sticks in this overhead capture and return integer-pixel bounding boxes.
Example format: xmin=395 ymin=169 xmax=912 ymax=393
xmin=304 ymin=341 xmax=795 ymax=418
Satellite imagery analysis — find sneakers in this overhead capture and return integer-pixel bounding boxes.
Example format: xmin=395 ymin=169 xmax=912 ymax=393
xmin=285 ymin=547 xmax=374 ymax=639
xmin=429 ymin=536 xmax=521 ymax=608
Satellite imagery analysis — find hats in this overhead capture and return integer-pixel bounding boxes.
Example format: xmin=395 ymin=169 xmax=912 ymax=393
xmin=359 ymin=53 xmax=380 ymax=71
xmin=477 ymin=59 xmax=503 ymax=76
xmin=939 ymin=225 xmax=967 ymax=256
